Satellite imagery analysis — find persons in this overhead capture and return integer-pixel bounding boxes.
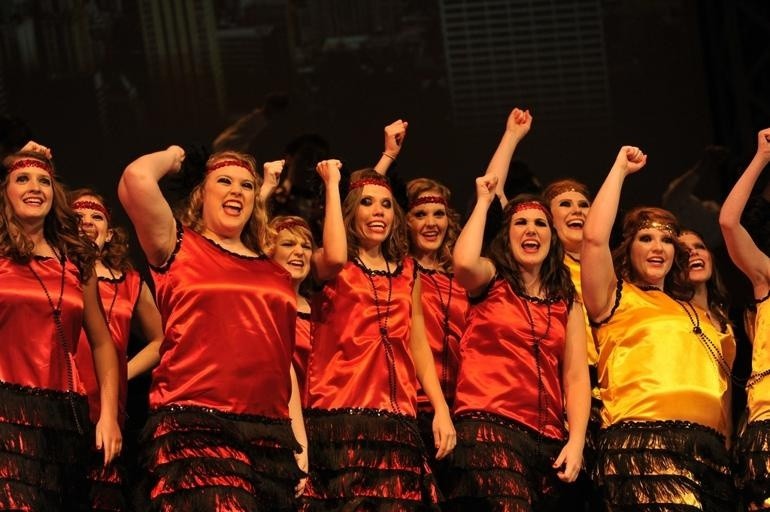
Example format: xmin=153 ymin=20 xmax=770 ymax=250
xmin=1 ymin=107 xmax=769 ymax=511
